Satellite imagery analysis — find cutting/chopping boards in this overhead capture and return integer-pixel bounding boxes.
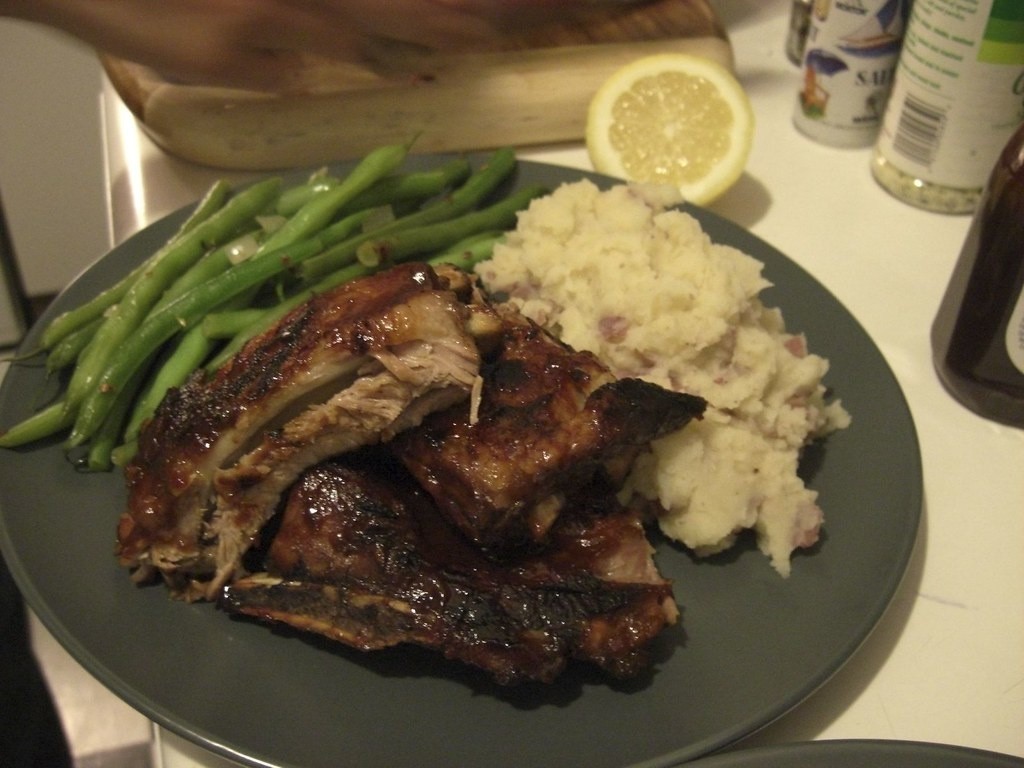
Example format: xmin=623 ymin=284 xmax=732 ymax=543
xmin=92 ymin=1 xmax=737 ymax=174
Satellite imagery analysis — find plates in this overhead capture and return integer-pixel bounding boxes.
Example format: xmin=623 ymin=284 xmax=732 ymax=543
xmin=1 ymin=152 xmax=925 ymax=768
xmin=670 ymin=737 xmax=1023 ymax=768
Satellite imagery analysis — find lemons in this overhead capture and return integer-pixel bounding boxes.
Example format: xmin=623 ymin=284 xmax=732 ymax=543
xmin=584 ymin=50 xmax=754 ymax=208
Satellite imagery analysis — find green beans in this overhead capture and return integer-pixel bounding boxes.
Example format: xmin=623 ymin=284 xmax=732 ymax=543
xmin=0 ymin=131 xmax=551 ymax=471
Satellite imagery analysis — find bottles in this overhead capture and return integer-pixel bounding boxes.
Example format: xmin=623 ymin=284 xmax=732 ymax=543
xmin=784 ymin=0 xmax=1024 ymax=430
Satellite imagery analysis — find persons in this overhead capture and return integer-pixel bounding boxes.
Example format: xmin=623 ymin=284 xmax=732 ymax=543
xmin=0 ymin=0 xmax=499 ymax=768
